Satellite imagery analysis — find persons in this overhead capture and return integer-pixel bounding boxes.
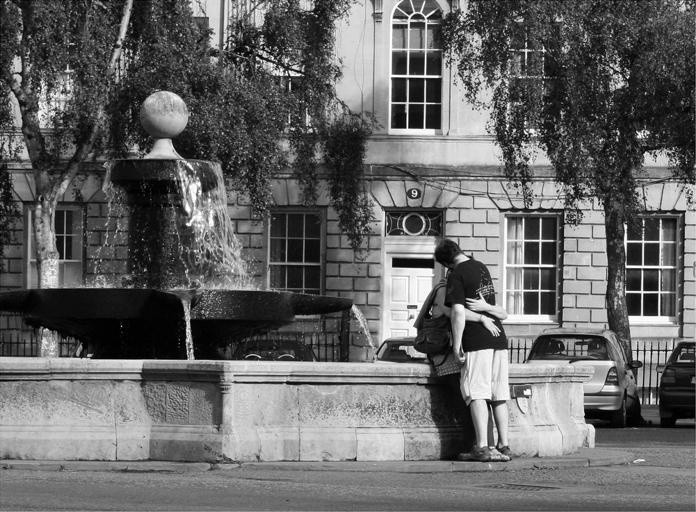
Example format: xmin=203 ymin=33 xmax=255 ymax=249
xmin=414 ymin=254 xmax=511 ymax=463
xmin=434 ymin=237 xmax=514 ymax=463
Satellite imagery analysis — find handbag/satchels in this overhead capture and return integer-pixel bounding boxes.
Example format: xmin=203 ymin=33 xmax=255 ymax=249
xmin=413 ymin=313 xmax=453 ymax=367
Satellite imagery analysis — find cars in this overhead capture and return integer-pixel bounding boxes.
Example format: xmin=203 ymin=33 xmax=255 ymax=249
xmin=233 ymin=339 xmax=319 ymax=362
xmin=372 ymin=337 xmax=430 ymax=364
xmin=522 ymin=326 xmax=642 ymax=428
xmin=656 ymin=342 xmax=696 ymax=427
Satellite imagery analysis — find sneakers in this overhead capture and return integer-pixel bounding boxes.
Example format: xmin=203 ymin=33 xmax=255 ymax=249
xmin=460 ymin=447 xmax=513 ymax=461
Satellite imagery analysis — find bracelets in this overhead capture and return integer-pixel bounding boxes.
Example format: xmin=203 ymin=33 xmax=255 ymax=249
xmin=479 ymin=313 xmax=483 ymax=324
